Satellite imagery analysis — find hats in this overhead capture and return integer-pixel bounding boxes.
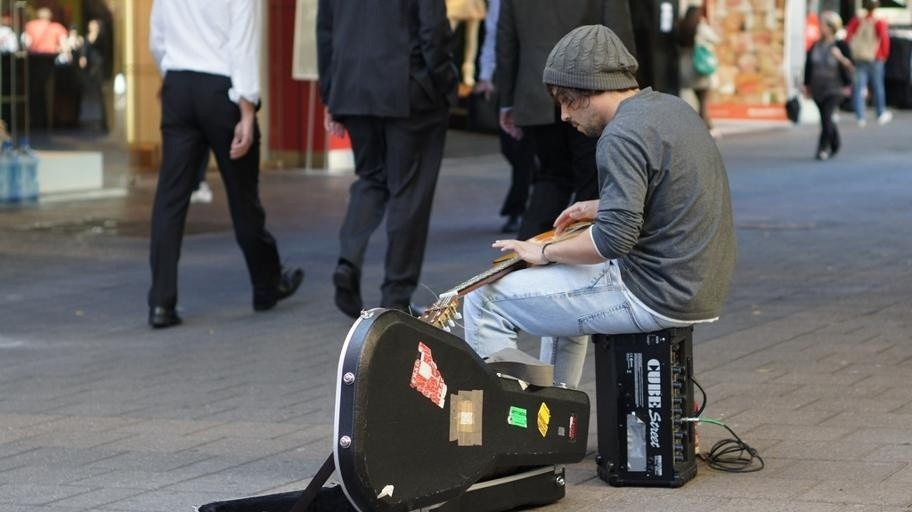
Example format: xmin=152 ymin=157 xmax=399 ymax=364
xmin=542 ymin=24 xmax=638 ymax=91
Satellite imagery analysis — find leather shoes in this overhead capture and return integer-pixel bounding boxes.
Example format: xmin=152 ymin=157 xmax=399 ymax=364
xmin=149 ymin=305 xmax=183 ymax=326
xmin=333 ymin=263 xmax=363 ymax=319
xmin=253 ymin=265 xmax=304 ymax=310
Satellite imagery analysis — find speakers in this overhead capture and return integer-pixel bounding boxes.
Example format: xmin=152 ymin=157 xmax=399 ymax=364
xmin=591 ymin=325 xmax=698 ymax=489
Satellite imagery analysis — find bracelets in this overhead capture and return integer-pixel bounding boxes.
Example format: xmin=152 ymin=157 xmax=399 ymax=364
xmin=541 ymin=240 xmax=557 ymax=264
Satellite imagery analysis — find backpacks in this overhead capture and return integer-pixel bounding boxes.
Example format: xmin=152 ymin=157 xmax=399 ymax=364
xmin=848 ymin=15 xmax=883 ymax=61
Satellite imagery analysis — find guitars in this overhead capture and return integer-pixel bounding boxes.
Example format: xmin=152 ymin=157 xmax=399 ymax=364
xmin=416 ymin=221 xmax=594 ymax=332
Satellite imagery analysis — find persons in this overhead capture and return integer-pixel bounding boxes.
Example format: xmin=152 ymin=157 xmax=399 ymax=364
xmin=0 ymin=8 xmax=119 ymax=138
xmin=848 ymin=1 xmax=892 ymax=126
xmin=462 ymin=22 xmax=736 ymax=400
xmin=498 ymin=1 xmax=644 ymax=259
xmin=314 ymin=1 xmax=466 ymax=321
xmin=475 ymin=1 xmax=679 ymax=234
xmin=678 ymin=4 xmax=719 ymax=136
xmin=800 ymin=9 xmax=854 ymax=159
xmin=146 ymin=0 xmax=303 ymax=329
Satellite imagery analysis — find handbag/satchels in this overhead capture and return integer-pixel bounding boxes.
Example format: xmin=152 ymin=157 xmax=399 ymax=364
xmin=694 ymin=46 xmax=717 ymax=76
